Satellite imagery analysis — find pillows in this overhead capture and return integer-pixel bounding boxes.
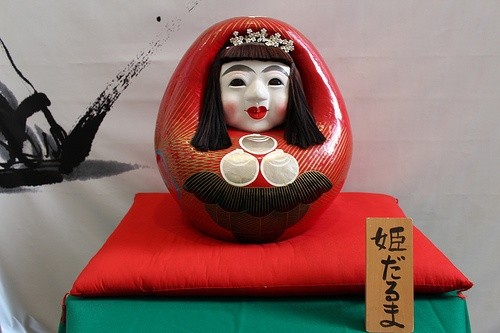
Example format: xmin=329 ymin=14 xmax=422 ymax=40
xmin=70 ymin=192 xmax=473 ymax=299
